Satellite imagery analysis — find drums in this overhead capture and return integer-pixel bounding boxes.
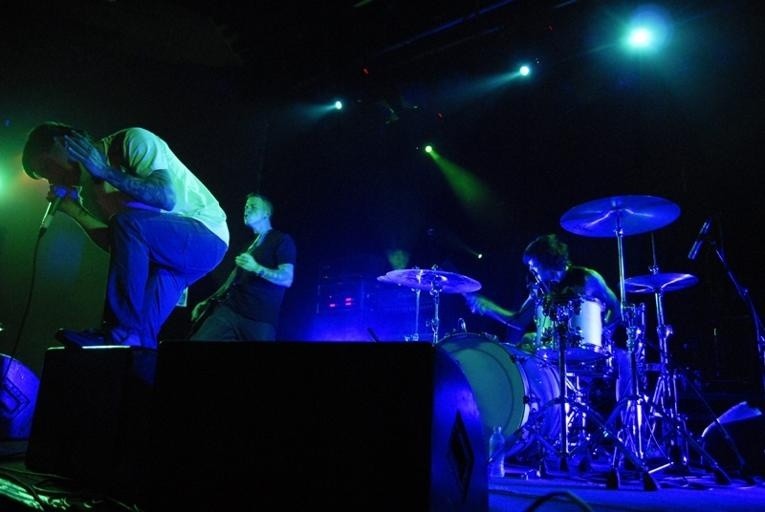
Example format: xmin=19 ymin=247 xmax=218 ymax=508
xmin=530 ymin=350 xmax=571 ymax=462
xmin=435 ymin=329 xmax=551 ymax=465
xmin=534 ymin=293 xmax=603 ymax=366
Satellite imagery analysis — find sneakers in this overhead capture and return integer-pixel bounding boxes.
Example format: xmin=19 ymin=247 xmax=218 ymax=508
xmin=54 ymin=319 xmax=142 ymax=352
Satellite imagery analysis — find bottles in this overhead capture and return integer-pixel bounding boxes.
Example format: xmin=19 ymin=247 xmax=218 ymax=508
xmin=488 ymin=426 xmax=505 ymax=478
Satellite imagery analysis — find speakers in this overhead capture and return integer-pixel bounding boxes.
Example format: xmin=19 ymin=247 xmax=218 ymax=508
xmin=0 ymin=352 xmax=40 ymax=441
xmin=24 ymin=337 xmax=490 ymax=512
xmin=37 ymin=182 xmax=69 ymax=238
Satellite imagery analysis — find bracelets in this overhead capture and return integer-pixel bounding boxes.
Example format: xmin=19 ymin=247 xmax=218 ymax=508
xmin=255 ymin=266 xmax=268 ymax=277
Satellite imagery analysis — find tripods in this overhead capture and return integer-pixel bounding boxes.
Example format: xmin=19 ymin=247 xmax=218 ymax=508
xmin=484 ymin=323 xmax=663 ymax=491
xmin=643 ymin=291 xmax=757 ymax=487
xmin=568 ymin=212 xmax=735 ymax=489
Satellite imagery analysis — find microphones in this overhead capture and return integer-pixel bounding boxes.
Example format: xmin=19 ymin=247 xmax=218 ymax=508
xmin=687 ymin=216 xmax=712 ymax=261
xmin=533 ymin=271 xmax=550 ymax=294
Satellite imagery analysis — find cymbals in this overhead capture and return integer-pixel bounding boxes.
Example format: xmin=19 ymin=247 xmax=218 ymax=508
xmin=380 ymin=276 xmax=432 ymax=291
xmin=619 ymin=274 xmax=697 ymax=296
xmin=560 ymin=194 xmax=680 ymax=238
xmin=386 ymin=268 xmax=482 ymax=294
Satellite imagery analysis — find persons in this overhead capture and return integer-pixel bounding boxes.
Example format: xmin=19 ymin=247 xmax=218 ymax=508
xmin=462 ymin=232 xmax=626 ymax=402
xmin=179 ymin=192 xmax=298 ymax=341
xmin=21 ymin=119 xmax=232 ymax=353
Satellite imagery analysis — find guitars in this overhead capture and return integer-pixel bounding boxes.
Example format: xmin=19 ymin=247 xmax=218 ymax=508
xmin=189 ymin=233 xmax=263 ymax=332
